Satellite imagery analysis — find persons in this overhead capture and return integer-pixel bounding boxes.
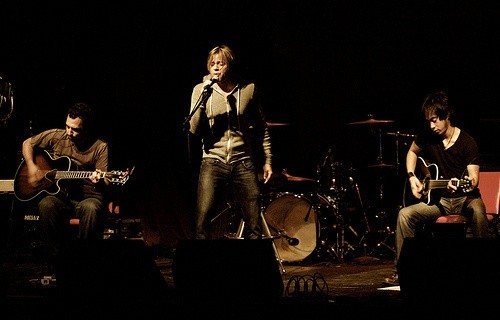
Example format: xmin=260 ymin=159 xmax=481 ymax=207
xmin=22 ymin=103 xmax=109 ymax=284
xmin=182 ymin=44 xmax=272 ymax=241
xmin=383 ymin=91 xmax=489 ymax=285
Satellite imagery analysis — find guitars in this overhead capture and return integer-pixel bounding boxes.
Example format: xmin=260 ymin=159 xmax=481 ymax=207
xmin=403 ymin=157 xmax=473 ymax=207
xmin=13 ymin=149 xmax=132 ymax=204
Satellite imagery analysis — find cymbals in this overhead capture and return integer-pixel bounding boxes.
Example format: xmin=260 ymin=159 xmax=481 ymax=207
xmin=345 ymin=119 xmax=395 ymax=125
xmin=283 ymin=173 xmax=315 ymax=182
xmin=266 ymin=122 xmax=289 ymax=126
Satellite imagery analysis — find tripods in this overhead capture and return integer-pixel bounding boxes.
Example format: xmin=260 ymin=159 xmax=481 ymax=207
xmin=323 ymin=127 xmax=402 ymax=263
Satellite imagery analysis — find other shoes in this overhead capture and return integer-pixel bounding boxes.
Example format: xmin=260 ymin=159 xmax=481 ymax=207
xmin=385 ymin=272 xmax=400 ymax=286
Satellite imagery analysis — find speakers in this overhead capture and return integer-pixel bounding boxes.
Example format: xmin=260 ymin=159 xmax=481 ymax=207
xmin=53 ymin=238 xmax=170 ymax=320
xmin=172 ymin=238 xmax=284 ymax=315
xmin=396 ymin=236 xmax=500 ymax=320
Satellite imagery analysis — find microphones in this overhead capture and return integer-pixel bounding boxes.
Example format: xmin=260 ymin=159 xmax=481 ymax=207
xmin=204 ymin=74 xmax=219 ymax=90
xmin=289 ymin=238 xmax=299 ymax=246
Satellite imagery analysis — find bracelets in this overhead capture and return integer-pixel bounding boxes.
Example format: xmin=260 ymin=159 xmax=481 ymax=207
xmin=199 ymin=103 xmax=206 ymax=109
xmin=407 ymin=172 xmax=416 ymax=179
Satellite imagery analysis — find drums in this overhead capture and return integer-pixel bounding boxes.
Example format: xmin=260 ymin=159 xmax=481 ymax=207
xmin=261 ymin=193 xmax=338 ymax=264
